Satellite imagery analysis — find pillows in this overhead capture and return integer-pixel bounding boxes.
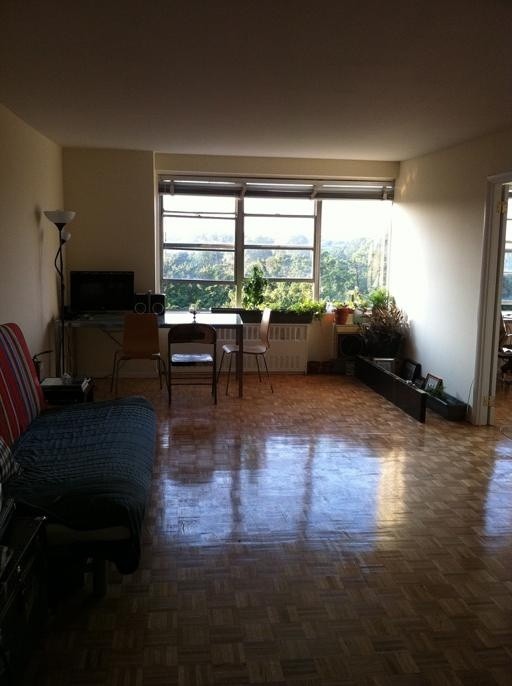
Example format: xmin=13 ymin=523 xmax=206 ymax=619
xmin=0 ymin=434 xmax=24 ymax=485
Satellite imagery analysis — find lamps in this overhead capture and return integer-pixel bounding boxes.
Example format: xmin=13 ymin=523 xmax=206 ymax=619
xmin=41 ymin=207 xmax=76 ymax=380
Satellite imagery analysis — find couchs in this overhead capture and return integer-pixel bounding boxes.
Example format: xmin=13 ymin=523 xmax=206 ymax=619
xmin=1 ymin=318 xmax=162 ymax=602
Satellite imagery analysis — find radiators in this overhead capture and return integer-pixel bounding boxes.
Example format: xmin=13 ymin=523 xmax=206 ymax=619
xmin=169 ymin=321 xmax=310 ymax=375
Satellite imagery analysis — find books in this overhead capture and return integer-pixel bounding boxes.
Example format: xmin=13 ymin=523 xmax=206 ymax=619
xmin=40 ymin=377 xmax=88 ymax=392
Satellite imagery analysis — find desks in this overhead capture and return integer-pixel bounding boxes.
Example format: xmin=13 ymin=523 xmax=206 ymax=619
xmin=1 ymin=494 xmax=52 ymax=685
xmin=46 ymin=310 xmax=245 ymax=408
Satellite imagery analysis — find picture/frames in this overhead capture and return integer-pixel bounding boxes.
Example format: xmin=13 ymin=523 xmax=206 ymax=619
xmin=401 ymin=357 xmax=422 ymax=384
xmin=412 ymin=375 xmax=426 ymax=389
xmin=422 ymin=372 xmax=442 ymax=397
xmin=371 ymin=357 xmax=396 ymax=375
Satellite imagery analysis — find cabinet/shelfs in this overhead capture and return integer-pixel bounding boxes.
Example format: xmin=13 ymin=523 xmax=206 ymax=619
xmin=38 ymin=376 xmax=96 ymax=407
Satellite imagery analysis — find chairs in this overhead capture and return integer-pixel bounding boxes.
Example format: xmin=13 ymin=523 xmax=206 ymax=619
xmin=109 ymin=312 xmax=171 ymax=404
xmin=209 ymin=307 xmax=275 ymax=399
xmin=163 ymin=321 xmax=220 ymax=409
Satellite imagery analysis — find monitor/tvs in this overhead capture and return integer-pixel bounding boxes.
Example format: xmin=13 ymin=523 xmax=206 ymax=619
xmin=70 ymin=271 xmax=134 ymax=310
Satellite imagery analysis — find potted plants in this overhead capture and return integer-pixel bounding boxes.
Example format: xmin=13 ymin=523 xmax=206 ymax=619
xmin=329 ymin=302 xmax=354 ymax=325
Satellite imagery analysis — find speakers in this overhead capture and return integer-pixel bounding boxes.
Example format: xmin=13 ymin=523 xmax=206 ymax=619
xmin=135 ymin=293 xmax=149 ymax=315
xmin=150 ymin=294 xmax=165 ymax=317
